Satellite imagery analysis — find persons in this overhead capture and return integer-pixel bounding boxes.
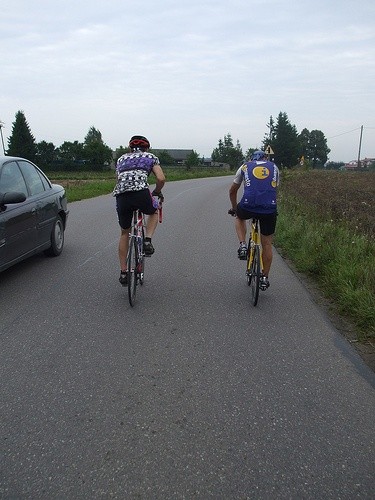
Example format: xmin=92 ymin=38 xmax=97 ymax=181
xmin=228 ymin=151 xmax=279 ymax=289
xmin=113 ymin=136 xmax=166 ymax=287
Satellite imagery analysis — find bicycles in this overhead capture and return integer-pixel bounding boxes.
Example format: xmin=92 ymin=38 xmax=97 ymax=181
xmin=228 ymin=209 xmax=267 ymax=307
xmin=121 ymin=192 xmax=165 ymax=307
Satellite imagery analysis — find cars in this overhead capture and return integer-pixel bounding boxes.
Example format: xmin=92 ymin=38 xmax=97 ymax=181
xmin=0 ymin=155 xmax=71 ymax=273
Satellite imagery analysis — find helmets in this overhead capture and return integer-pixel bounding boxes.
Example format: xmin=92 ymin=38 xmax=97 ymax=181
xmin=129 ymin=136 xmax=150 ymax=152
xmin=251 ymin=150 xmax=266 ymax=161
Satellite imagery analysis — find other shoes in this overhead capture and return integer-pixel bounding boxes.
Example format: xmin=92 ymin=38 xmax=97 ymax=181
xmin=260 ymin=276 xmax=269 ymax=291
xmin=143 ymin=237 xmax=154 ymax=254
xmin=238 ymin=241 xmax=247 ymax=257
xmin=119 ymin=270 xmax=128 ymax=283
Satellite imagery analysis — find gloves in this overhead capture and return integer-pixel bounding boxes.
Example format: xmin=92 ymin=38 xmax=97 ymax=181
xmin=228 ymin=209 xmax=236 ymax=216
xmin=152 ymin=192 xmax=164 ymax=202
